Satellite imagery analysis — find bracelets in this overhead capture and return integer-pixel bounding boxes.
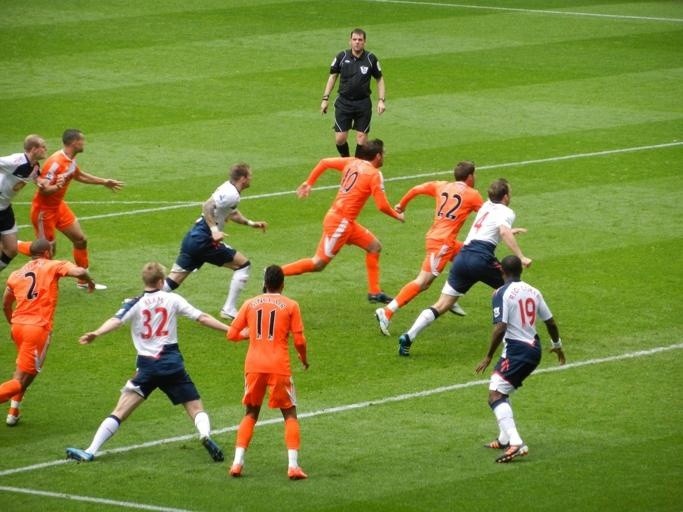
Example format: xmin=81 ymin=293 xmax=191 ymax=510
xmin=548 ymin=338 xmax=562 ymax=350
xmin=208 ymin=225 xmax=219 ymax=233
xmin=376 ymin=97 xmax=386 ymax=102
xmin=321 ymin=94 xmax=330 ymax=100
xmin=243 ymin=218 xmax=249 ymax=226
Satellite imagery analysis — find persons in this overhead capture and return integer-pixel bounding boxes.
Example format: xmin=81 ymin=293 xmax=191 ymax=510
xmin=0 ymin=132 xmax=48 ymax=273
xmin=261 ymin=137 xmax=409 ymax=305
xmin=16 ymin=128 xmax=126 ymax=290
xmin=0 ymin=239 xmax=95 ymax=427
xmin=316 ymin=25 xmax=387 ymax=159
xmin=120 ymin=161 xmax=267 ymax=322
xmin=397 ymin=175 xmax=534 ymax=358
xmin=372 ymin=159 xmax=485 ymax=338
xmin=224 ymin=263 xmax=311 ymax=481
xmin=64 ymin=260 xmax=232 ymax=465
xmin=470 ymin=254 xmax=567 ymax=465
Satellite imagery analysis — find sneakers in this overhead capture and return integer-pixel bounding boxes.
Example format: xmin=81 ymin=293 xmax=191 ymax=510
xmin=449 ymin=302 xmax=466 ymax=317
xmin=66 ymin=448 xmax=94 ymax=462
xmin=6 ymin=414 xmax=19 ymax=427
xmin=220 ymin=308 xmax=238 ymax=320
xmin=398 ymin=333 xmax=413 ymax=356
xmin=202 ymin=436 xmax=224 ymax=462
xmin=375 ymin=308 xmax=392 ymax=336
xmin=229 ymin=464 xmax=243 ymax=477
xmin=287 ymin=465 xmax=307 ymax=480
xmin=77 ymin=282 xmax=107 ymax=290
xmin=484 ymin=438 xmax=528 ymax=463
xmin=368 ymin=289 xmax=393 ymax=305
